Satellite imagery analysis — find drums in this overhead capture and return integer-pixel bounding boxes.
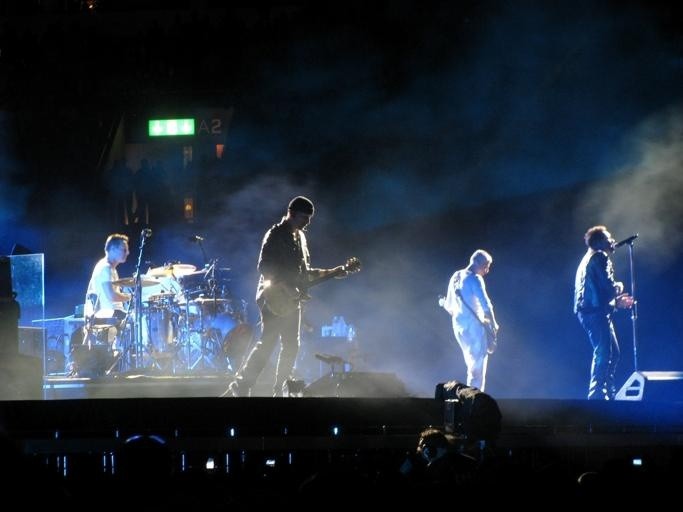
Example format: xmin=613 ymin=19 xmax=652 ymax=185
xmin=120 ymin=310 xmax=228 ymax=374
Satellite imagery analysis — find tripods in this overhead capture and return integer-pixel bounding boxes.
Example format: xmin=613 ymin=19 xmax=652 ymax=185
xmin=105 ymin=273 xmax=234 ymax=376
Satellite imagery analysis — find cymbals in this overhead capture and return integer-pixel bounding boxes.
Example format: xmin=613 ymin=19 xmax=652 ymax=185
xmin=178 ymin=299 xmax=228 ymax=304
xmin=151 ymin=264 xmax=230 ymax=277
xmin=112 ymin=277 xmax=160 ymax=287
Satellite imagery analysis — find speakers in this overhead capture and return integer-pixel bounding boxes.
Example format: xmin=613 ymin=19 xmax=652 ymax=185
xmin=614 ymin=371 xmax=683 ymax=402
xmin=303 ymin=372 xmax=408 ymax=398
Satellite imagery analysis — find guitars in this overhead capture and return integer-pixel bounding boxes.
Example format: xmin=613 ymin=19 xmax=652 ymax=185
xmin=263 ymin=257 xmax=362 ymax=316
xmin=436 ymin=293 xmax=497 ymax=355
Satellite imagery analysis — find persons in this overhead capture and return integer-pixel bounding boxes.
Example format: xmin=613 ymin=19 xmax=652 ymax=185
xmin=81 ymin=233 xmax=135 ymax=357
xmin=447 ymin=249 xmax=501 ymax=394
xmin=571 ymin=226 xmax=635 ymax=401
xmin=227 ymin=194 xmax=349 ymax=396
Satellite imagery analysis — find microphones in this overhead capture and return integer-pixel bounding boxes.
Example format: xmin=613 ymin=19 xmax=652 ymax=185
xmin=141 ymin=228 xmax=152 ymax=237
xmin=191 ymin=236 xmax=204 ymax=241
xmin=615 ymin=234 xmax=638 ymax=247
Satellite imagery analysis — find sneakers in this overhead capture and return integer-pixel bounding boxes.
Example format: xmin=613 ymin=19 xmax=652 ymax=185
xmin=229 ymin=377 xmax=252 ymax=397
